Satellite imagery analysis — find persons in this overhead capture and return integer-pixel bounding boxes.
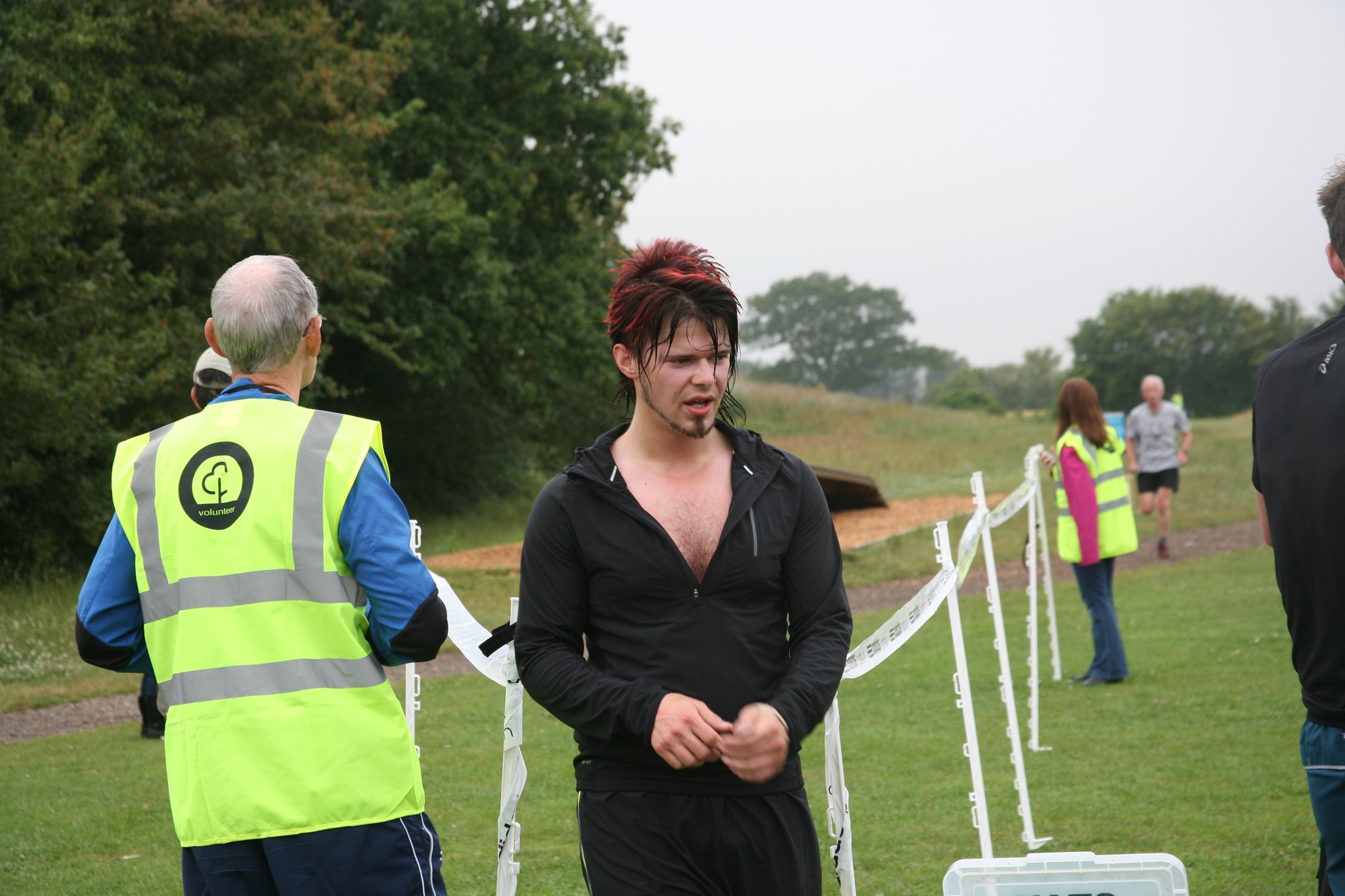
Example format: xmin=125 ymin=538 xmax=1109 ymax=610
xmin=513 ymin=236 xmax=855 ymax=896
xmin=1037 ymin=377 xmax=1139 ymax=690
xmin=133 ymin=347 xmax=231 ymax=739
xmin=1127 ymin=373 xmax=1193 ymax=559
xmin=74 ymin=256 xmax=450 ymax=896
xmin=1245 ymin=168 xmax=1345 ymax=896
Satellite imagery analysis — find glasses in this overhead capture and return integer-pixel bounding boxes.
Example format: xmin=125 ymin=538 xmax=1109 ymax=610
xmin=302 ymin=314 xmax=327 ymax=337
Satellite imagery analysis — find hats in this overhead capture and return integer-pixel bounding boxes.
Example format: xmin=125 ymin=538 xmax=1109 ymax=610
xmin=193 ymin=347 xmax=234 ymax=389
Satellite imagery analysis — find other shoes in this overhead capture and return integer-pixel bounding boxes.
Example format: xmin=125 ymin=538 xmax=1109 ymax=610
xmin=1070 ymin=670 xmax=1090 ymax=680
xmin=1083 ymin=677 xmax=1121 ymax=685
xmin=1157 ymin=541 xmax=1170 ymax=558
